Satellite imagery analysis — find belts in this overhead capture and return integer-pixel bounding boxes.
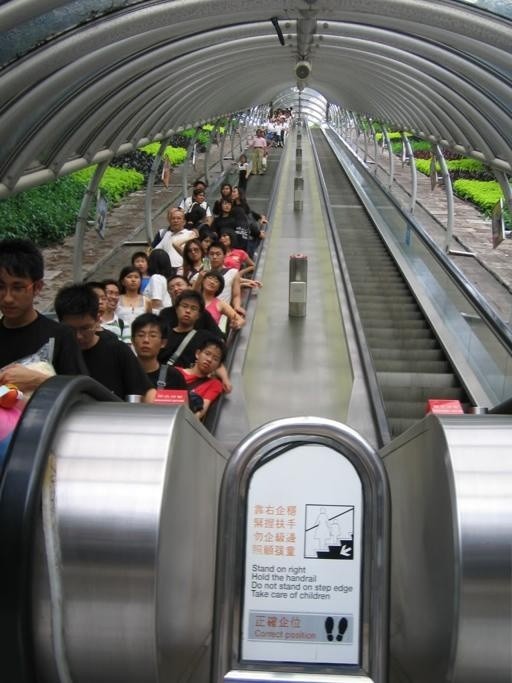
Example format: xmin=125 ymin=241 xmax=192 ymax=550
xmin=254 ymin=146 xmax=262 ymax=148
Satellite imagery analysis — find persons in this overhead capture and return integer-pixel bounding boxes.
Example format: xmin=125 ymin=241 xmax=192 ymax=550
xmin=190 ymin=241 xmax=246 ymax=318
xmin=101 ymin=280 xmax=134 ymax=349
xmin=157 ymin=289 xmax=231 ymax=394
xmin=260 ymin=130 xmax=272 ymax=171
xmin=114 ymin=267 xmax=152 ymax=358
xmin=211 ymin=198 xmax=235 ymax=237
xmin=245 ymin=128 xmax=267 ymax=175
xmin=213 ymin=184 xmax=267 ymax=225
xmin=55 ymin=284 xmax=157 ymax=403
xmin=132 ymin=252 xmax=150 ymax=295
xmin=131 ymin=312 xmax=187 ymax=390
xmin=159 ymin=276 xmax=242 ymax=350
xmin=149 ymin=210 xmax=197 ymax=277
xmin=187 ymin=189 xmax=205 ymax=214
xmin=172 ymin=229 xmax=219 ymax=271
xmin=266 ymin=108 xmax=292 ymax=148
xmin=216 ymin=228 xmax=263 ymax=288
xmin=143 ymin=249 xmax=173 ymax=316
xmin=174 ymin=339 xmax=228 ymax=419
xmin=201 ymin=272 xmax=246 ymax=330
xmin=151 ymin=208 xmax=187 ymax=249
xmin=220 ymin=205 xmax=265 ymax=259
xmin=184 ymin=206 xmax=219 ymax=245
xmin=0 ymin=238 xmax=91 ymax=392
xmin=231 ymin=186 xmax=250 ymax=216
xmin=177 ymin=239 xmax=258 ymax=289
xmin=84 ymin=282 xmax=118 ymax=339
xmin=234 ymin=155 xmax=252 ymax=189
xmin=178 ymin=181 xmax=213 ymax=226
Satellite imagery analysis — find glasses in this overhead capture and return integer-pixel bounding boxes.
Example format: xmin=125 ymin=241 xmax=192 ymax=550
xmin=72 ymin=319 xmax=97 ymax=335
xmin=168 ymin=281 xmax=188 ymax=294
xmin=97 ymin=296 xmax=108 ymax=303
xmin=186 ymin=246 xmax=200 ymax=253
xmin=0 ymin=278 xmax=39 ymax=300
xmin=177 ymin=302 xmax=201 ymax=313
xmin=133 ymin=330 xmax=162 ymax=341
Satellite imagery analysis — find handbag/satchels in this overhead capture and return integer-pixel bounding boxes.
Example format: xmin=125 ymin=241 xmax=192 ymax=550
xmin=261 ymin=156 xmax=268 ymax=171
xmin=246 ymin=239 xmax=256 ymax=259
